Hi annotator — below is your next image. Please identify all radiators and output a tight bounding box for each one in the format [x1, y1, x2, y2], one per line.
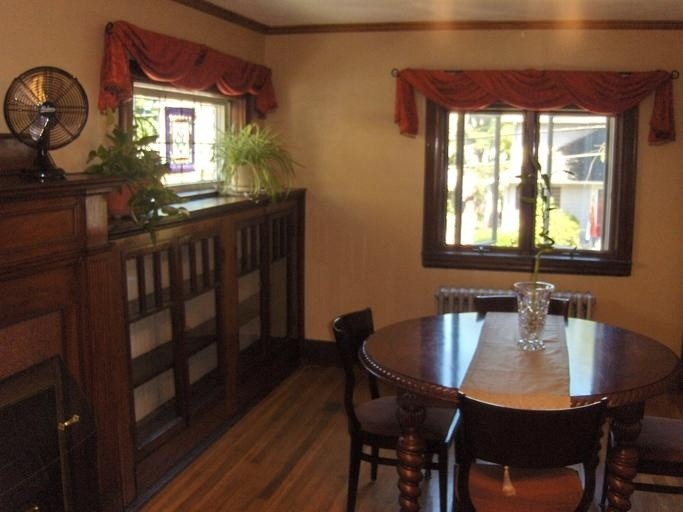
[433, 286, 598, 320]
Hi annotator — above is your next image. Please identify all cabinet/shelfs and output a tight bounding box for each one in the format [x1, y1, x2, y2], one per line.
[0, 174, 136, 510]
[122, 187, 309, 502]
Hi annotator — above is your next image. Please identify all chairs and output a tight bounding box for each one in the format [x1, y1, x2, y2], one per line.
[474, 294, 568, 316]
[454, 388, 607, 511]
[599, 413, 682, 512]
[332, 307, 464, 512]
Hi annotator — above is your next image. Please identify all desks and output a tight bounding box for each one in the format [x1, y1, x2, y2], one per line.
[358, 310, 683, 512]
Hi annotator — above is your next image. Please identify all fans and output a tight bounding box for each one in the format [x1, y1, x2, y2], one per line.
[3, 65, 89, 183]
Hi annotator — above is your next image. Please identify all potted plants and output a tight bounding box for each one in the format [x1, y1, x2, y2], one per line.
[514, 120, 562, 350]
[82, 101, 187, 246]
[208, 120, 307, 206]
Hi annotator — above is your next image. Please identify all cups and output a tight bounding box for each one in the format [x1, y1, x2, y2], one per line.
[513, 281, 555, 352]
